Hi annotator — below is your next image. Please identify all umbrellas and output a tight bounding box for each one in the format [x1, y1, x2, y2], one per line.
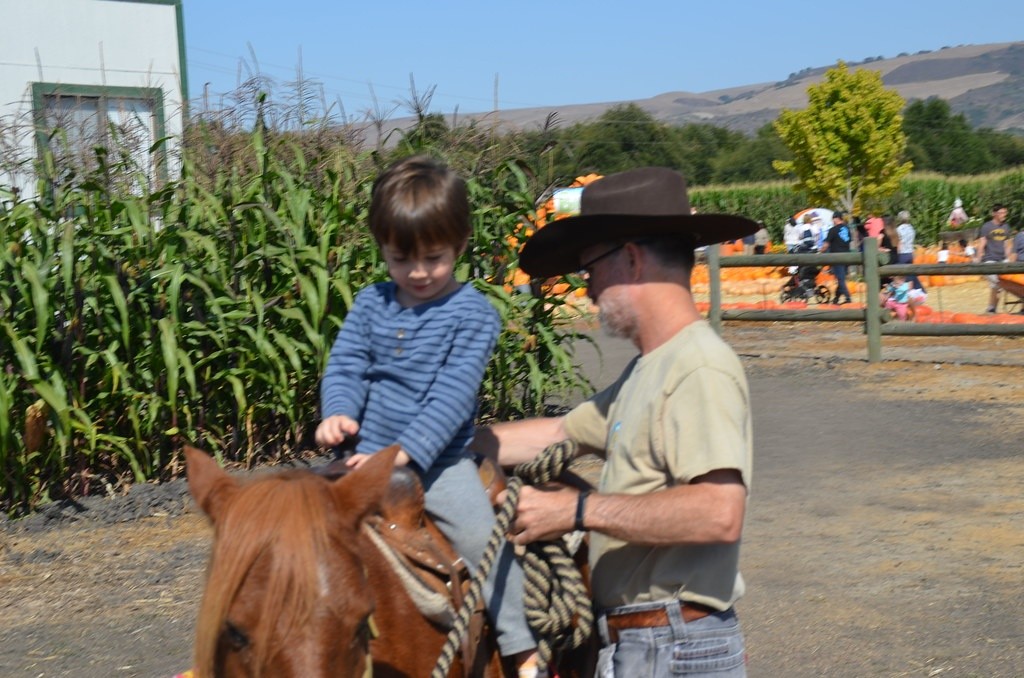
[786, 207, 836, 228]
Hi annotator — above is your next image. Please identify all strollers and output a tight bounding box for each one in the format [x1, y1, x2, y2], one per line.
[780, 240, 832, 304]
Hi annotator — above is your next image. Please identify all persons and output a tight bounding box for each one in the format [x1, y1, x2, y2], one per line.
[313, 153, 554, 678]
[755, 199, 1024, 322]
[470, 166, 764, 678]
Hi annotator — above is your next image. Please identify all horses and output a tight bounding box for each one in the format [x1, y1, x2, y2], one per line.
[183, 444, 599, 678]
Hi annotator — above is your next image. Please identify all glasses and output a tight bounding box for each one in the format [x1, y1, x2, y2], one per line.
[563, 248, 621, 286]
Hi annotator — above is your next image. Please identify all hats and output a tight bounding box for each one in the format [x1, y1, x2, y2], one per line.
[516, 166, 755, 279]
[833, 211, 843, 219]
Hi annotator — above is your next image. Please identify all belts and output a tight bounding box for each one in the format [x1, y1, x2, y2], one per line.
[606, 601, 716, 644]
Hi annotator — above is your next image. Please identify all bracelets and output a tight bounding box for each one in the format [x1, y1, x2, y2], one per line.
[576, 490, 592, 531]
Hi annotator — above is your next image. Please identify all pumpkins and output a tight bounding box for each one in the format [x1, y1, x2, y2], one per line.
[691, 242, 1024, 323]
[481, 174, 603, 295]
